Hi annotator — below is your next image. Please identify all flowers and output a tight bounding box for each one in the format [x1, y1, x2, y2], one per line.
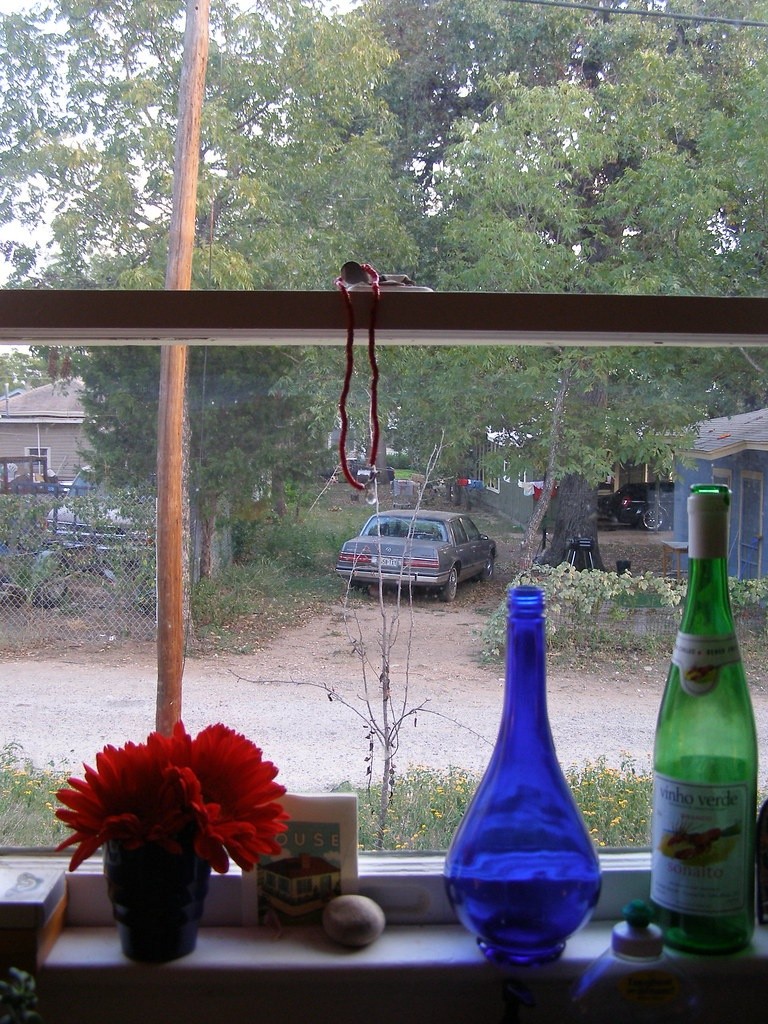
[55, 720, 291, 875]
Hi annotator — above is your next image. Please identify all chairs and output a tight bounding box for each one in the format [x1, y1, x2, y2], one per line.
[391, 480, 415, 510]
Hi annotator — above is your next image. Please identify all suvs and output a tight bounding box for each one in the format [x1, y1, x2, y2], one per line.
[608, 481, 674, 532]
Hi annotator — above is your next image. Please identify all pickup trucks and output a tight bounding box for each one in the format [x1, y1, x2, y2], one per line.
[319, 457, 395, 482]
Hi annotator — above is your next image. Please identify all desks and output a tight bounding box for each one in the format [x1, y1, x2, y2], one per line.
[661, 541, 688, 579]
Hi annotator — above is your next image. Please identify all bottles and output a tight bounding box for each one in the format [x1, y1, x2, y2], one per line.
[647, 484, 760, 956]
[442, 587, 603, 965]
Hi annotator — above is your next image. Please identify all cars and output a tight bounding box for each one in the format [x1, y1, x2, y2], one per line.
[335, 509, 497, 602]
[43, 463, 159, 568]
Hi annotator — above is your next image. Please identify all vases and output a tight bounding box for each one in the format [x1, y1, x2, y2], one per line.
[103, 841, 210, 965]
